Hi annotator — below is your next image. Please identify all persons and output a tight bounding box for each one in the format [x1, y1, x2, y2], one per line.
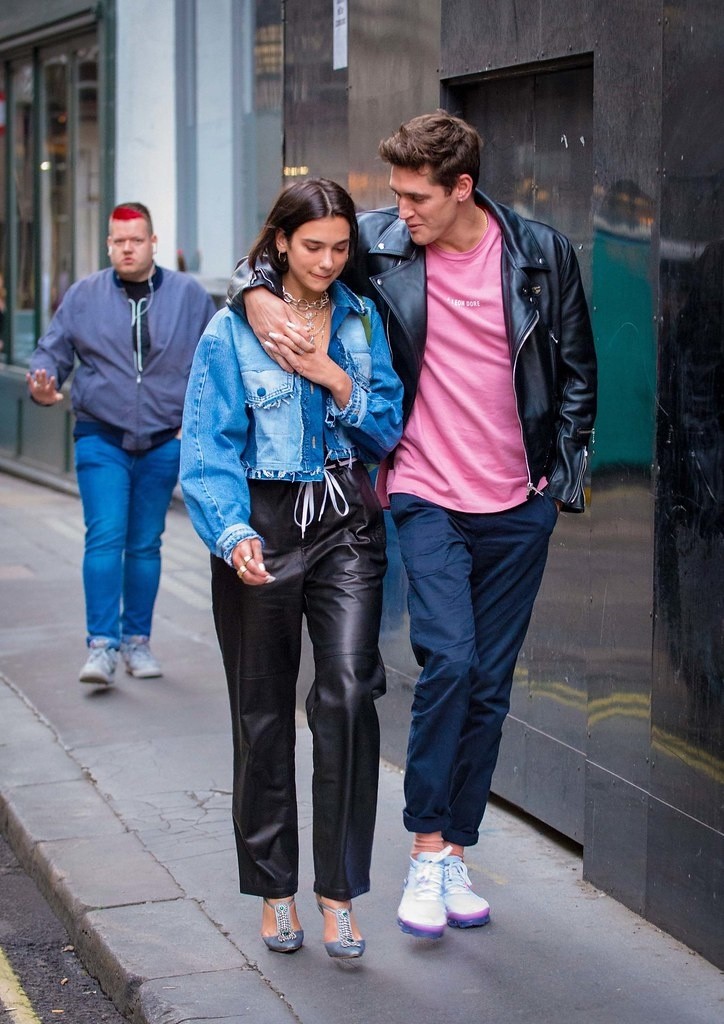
[177, 177, 403, 958]
[26, 203, 217, 683]
[225, 113, 598, 938]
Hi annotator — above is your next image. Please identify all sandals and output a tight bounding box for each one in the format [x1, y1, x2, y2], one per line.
[261, 894, 304, 953]
[314, 893, 366, 960]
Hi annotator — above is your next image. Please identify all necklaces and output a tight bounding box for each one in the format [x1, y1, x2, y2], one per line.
[283, 283, 329, 348]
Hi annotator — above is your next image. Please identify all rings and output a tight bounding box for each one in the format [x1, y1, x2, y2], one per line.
[244, 555, 251, 565]
[240, 566, 247, 573]
[237, 571, 243, 577]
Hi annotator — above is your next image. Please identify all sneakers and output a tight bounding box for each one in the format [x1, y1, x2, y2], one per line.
[396, 854, 448, 940]
[443, 854, 492, 928]
[118, 634, 165, 679]
[78, 642, 119, 685]
